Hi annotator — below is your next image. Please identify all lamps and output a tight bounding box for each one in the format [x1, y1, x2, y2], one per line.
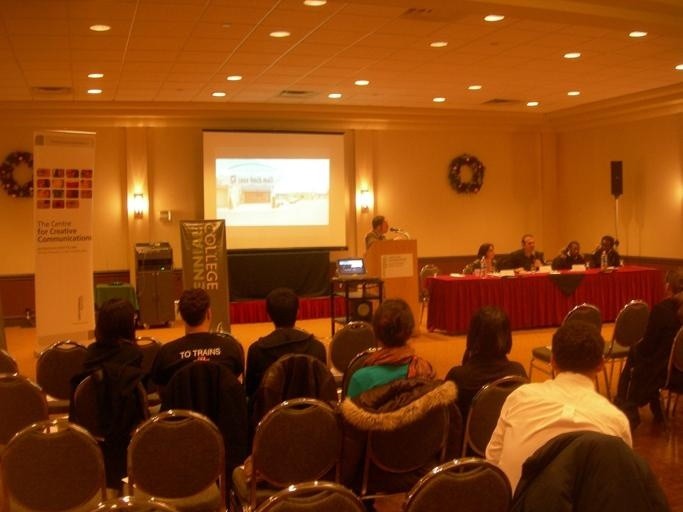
[352, 79, 370, 86]
[429, 40, 448, 48]
[565, 90, 581, 97]
[211, 90, 227, 97]
[327, 93, 343, 100]
[482, 14, 505, 23]
[86, 88, 103, 95]
[304, 0, 328, 7]
[225, 74, 243, 82]
[269, 31, 291, 38]
[672, 63, 683, 72]
[88, 22, 112, 33]
[561, 51, 582, 60]
[526, 100, 540, 108]
[87, 72, 104, 79]
[628, 30, 647, 39]
[467, 84, 481, 91]
[132, 193, 144, 220]
[359, 189, 370, 214]
[432, 96, 446, 104]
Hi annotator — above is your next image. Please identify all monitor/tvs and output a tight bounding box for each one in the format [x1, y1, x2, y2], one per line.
[338, 259, 366, 274]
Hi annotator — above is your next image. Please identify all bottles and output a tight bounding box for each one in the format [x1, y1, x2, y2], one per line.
[480, 256, 486, 277]
[601, 251, 607, 269]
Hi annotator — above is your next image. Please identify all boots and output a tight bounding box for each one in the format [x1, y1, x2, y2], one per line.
[651, 393, 667, 421]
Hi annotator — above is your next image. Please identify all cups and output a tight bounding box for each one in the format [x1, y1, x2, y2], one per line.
[531, 267, 536, 275]
[472, 269, 481, 278]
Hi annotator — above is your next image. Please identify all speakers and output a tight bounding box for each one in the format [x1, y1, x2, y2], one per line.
[610, 160, 624, 196]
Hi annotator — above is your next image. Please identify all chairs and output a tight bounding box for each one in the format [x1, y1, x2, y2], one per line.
[329, 321, 376, 384]
[360, 403, 450, 496]
[459, 374, 532, 474]
[602, 299, 649, 391]
[657, 325, 683, 418]
[254, 481, 366, 512]
[0, 373, 48, 445]
[136, 336, 161, 373]
[72, 370, 150, 442]
[160, 359, 246, 450]
[0, 420, 107, 512]
[127, 409, 226, 512]
[255, 354, 338, 416]
[229, 397, 341, 512]
[528, 304, 610, 401]
[402, 457, 512, 512]
[341, 347, 383, 399]
[35, 339, 88, 400]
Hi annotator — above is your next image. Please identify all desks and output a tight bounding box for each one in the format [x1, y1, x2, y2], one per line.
[424, 263, 665, 334]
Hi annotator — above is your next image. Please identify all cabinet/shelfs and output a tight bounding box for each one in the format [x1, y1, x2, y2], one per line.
[365, 237, 421, 330]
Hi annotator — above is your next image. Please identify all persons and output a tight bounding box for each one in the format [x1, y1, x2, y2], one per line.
[347, 299, 437, 399]
[552, 241, 585, 270]
[486, 321, 633, 496]
[613, 268, 683, 427]
[510, 234, 546, 271]
[365, 215, 389, 250]
[593, 236, 620, 267]
[83, 298, 159, 391]
[243, 287, 328, 398]
[444, 305, 528, 414]
[469, 242, 500, 274]
[151, 288, 245, 402]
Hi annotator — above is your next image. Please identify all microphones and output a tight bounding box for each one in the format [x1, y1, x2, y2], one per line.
[390, 227, 402, 231]
